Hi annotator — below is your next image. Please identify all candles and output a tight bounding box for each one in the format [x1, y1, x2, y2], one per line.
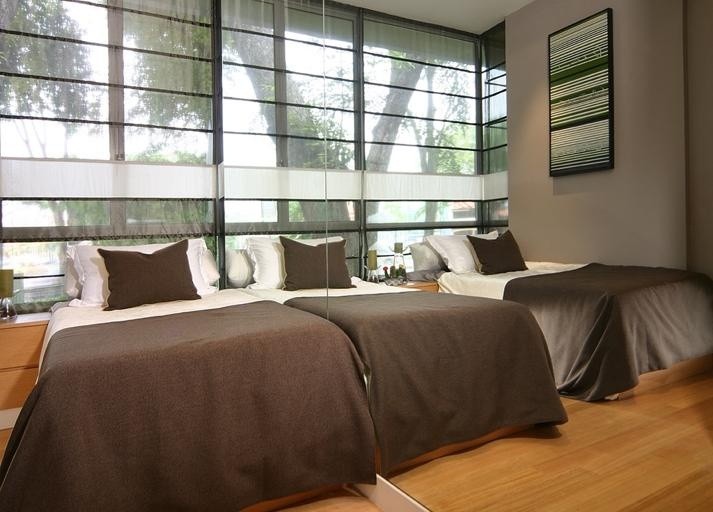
[0, 270, 13, 299]
[367, 250, 377, 270]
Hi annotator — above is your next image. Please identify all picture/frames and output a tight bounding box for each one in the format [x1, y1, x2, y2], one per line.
[548, 7, 614, 177]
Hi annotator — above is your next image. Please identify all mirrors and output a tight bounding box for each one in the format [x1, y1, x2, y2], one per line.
[211, 1, 713, 512]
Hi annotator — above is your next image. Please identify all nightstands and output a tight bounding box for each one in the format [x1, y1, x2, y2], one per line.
[0, 313, 51, 462]
[390, 279, 440, 293]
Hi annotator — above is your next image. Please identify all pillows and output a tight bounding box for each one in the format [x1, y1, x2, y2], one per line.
[226, 235, 356, 291]
[412, 229, 527, 275]
[61, 239, 222, 313]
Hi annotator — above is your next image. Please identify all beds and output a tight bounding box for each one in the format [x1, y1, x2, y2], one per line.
[442, 260, 713, 399]
[0, 292, 379, 512]
[228, 280, 568, 478]
